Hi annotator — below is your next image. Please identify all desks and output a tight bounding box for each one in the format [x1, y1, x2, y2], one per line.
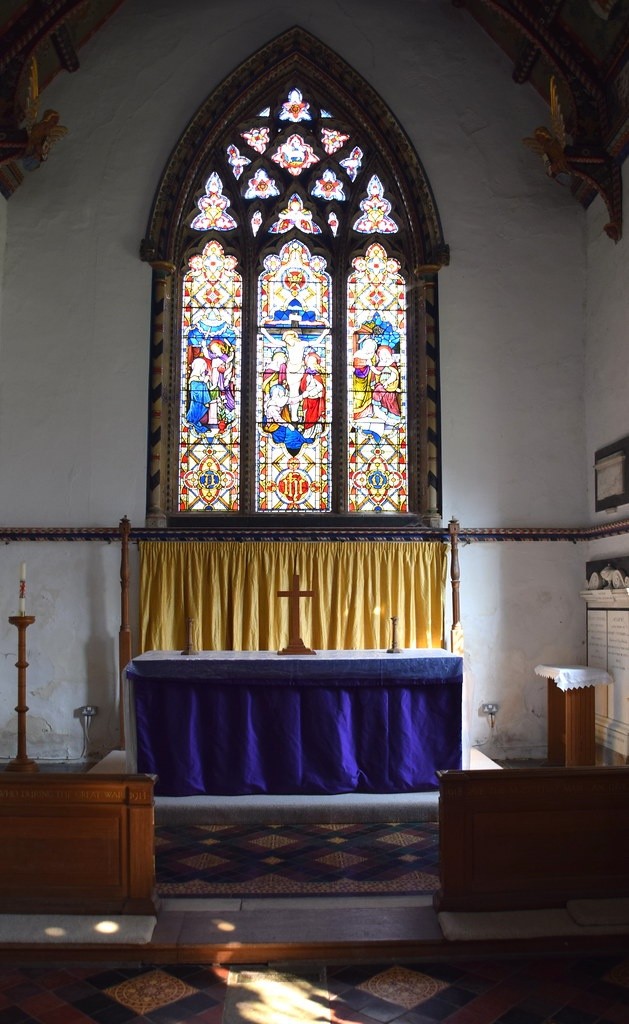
[122, 648, 464, 798]
[534, 665, 616, 768]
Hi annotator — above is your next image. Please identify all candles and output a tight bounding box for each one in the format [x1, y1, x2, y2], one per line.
[20, 561, 26, 612]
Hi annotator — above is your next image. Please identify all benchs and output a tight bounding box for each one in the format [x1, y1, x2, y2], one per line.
[0, 771, 160, 943]
[434, 769, 629, 940]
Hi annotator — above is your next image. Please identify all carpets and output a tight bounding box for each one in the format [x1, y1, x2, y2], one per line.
[154, 823, 442, 897]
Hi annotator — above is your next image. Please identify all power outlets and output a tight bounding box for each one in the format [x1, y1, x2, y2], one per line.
[482, 704, 499, 713]
[83, 705, 97, 715]
